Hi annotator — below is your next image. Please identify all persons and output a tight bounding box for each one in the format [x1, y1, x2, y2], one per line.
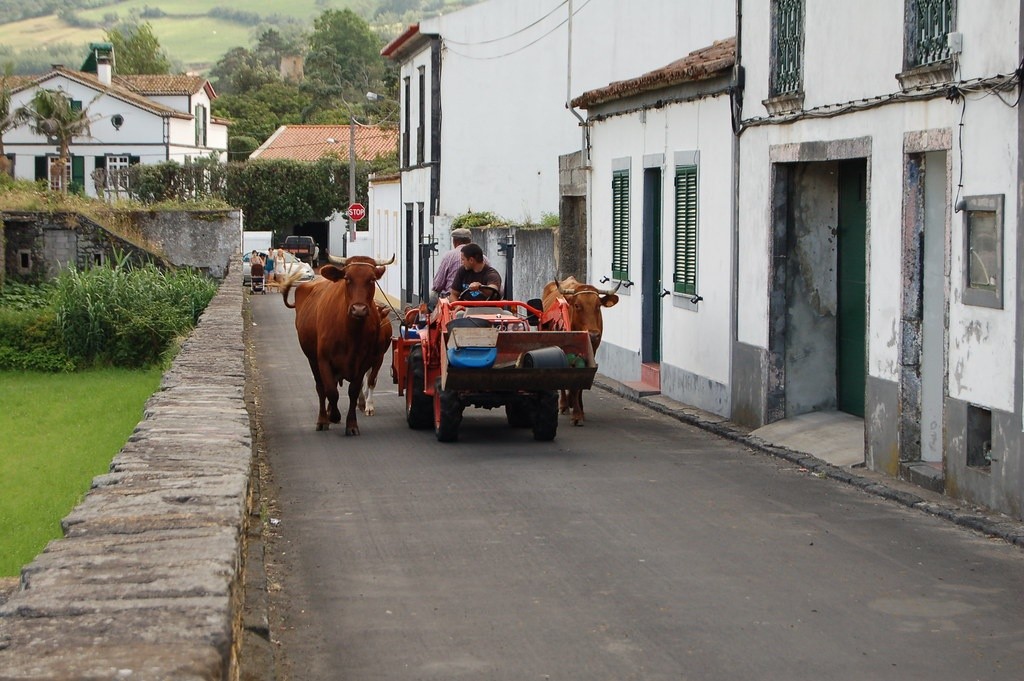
[264, 248, 276, 293]
[250, 250, 264, 294]
[275, 249, 286, 293]
[312, 243, 319, 269]
[427, 228, 491, 315]
[449, 244, 501, 319]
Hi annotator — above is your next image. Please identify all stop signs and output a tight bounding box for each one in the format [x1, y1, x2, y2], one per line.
[348, 202, 366, 222]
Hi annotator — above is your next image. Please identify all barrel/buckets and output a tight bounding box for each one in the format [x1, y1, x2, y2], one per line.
[445, 346, 499, 368]
[523, 346, 569, 369]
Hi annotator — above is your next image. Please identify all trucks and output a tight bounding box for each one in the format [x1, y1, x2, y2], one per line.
[280, 235, 320, 268]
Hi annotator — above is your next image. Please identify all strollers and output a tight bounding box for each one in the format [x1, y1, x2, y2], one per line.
[249, 263, 267, 295]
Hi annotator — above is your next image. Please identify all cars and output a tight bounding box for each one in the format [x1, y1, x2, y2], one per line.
[242, 249, 315, 286]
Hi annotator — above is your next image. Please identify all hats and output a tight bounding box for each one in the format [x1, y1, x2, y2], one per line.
[451, 228, 471, 238]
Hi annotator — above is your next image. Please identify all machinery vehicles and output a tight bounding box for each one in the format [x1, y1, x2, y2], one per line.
[389, 235, 599, 443]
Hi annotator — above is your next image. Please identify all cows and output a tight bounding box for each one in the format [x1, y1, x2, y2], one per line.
[266, 253, 398, 437]
[541, 275, 622, 427]
